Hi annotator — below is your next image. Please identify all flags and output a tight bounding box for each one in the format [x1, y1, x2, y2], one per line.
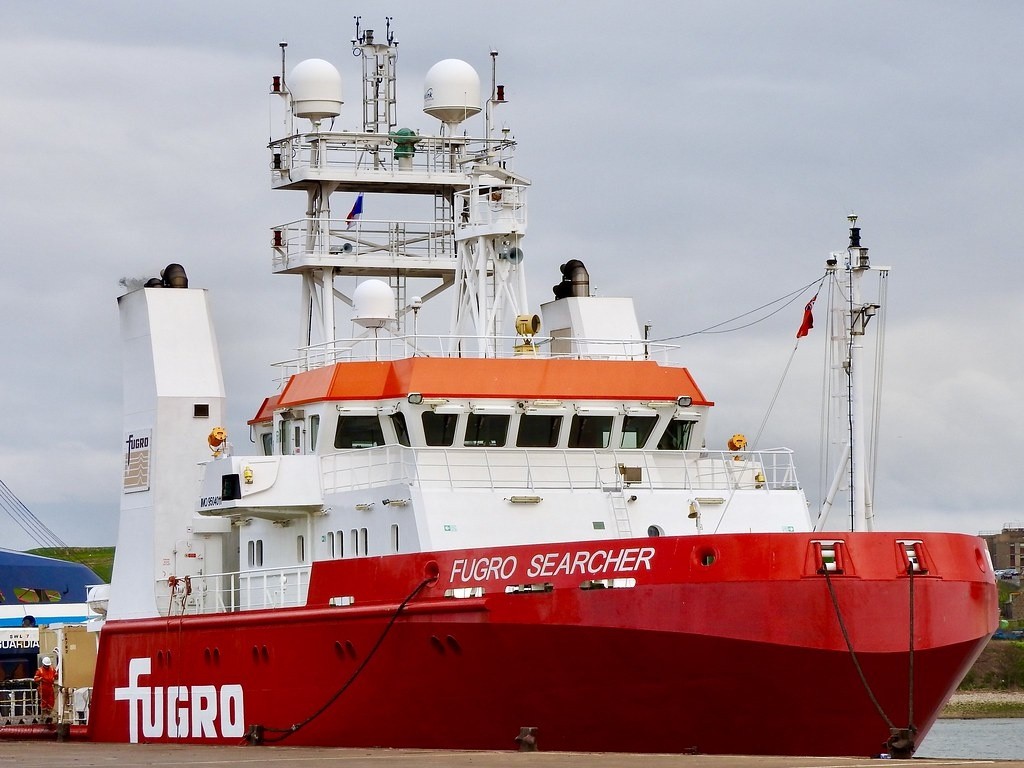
[345, 192, 363, 230]
[796, 292, 819, 338]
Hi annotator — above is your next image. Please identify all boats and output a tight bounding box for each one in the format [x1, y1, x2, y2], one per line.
[0, 11, 1001, 755]
[0, 480, 108, 688]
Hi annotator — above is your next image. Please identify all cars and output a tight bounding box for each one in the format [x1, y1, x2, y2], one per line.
[994, 568, 1020, 580]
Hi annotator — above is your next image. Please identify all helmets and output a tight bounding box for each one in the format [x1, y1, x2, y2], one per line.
[42, 657, 52, 666]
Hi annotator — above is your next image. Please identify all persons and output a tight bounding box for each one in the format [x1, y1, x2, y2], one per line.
[34, 657, 58, 723]
